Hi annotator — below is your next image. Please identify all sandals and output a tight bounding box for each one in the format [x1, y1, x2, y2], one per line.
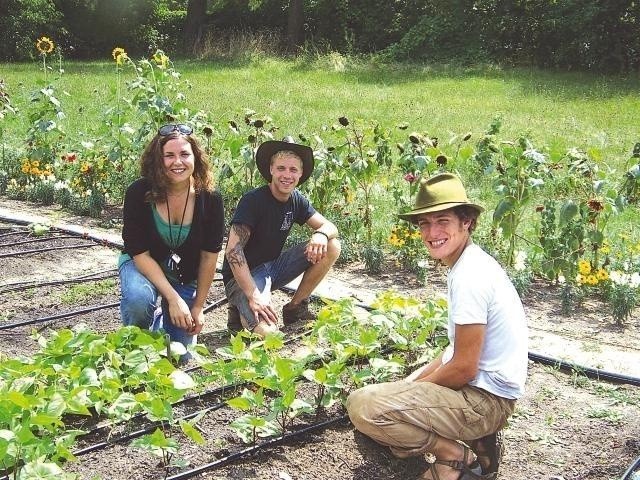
[472, 427, 505, 478]
[416, 444, 482, 480]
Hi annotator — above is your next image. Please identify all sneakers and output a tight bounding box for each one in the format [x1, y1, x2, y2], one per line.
[281, 300, 319, 326]
[226, 304, 243, 333]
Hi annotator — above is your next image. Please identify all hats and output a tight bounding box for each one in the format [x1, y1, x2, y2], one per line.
[255, 139, 315, 189]
[395, 171, 487, 222]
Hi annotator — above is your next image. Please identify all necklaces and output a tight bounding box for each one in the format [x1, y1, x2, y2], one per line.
[163, 183, 192, 273]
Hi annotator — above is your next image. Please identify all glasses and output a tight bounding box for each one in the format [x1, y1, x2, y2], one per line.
[157, 123, 193, 136]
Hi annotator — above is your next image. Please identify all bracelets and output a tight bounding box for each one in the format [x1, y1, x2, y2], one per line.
[310, 231, 330, 240]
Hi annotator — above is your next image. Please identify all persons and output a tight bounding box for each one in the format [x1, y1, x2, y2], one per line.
[339, 171, 526, 480]
[116, 123, 227, 356]
[218, 132, 343, 341]
